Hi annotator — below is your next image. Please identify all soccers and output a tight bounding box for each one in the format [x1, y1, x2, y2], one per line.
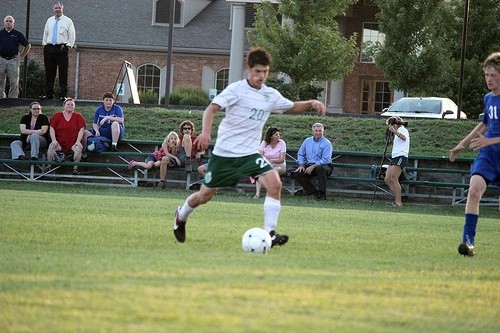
[242, 227, 272, 254]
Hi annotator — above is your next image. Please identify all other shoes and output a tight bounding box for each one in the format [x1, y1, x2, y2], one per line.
[459, 243, 475, 256]
[45, 95, 53, 99]
[60, 96, 66, 100]
[111, 145, 118, 151]
[39, 167, 51, 173]
[317, 195, 326, 200]
[315, 192, 321, 198]
[73, 169, 80, 174]
[185, 162, 192, 173]
[81, 157, 87, 161]
[158, 182, 165, 189]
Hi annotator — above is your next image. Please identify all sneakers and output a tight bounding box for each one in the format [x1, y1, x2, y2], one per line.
[173, 206, 186, 242]
[270, 230, 288, 248]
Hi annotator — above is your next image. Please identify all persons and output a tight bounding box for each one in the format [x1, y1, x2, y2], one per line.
[292, 123, 335, 200]
[39, 2, 76, 100]
[253, 128, 287, 199]
[128, 120, 208, 189]
[449, 53, 500, 255]
[10, 102, 51, 161]
[83, 92, 126, 159]
[45, 97, 86, 175]
[173, 48, 326, 248]
[384, 116, 409, 208]
[0, 16, 31, 98]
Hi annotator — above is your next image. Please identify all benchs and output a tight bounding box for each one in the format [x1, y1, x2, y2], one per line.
[0, 132, 500, 208]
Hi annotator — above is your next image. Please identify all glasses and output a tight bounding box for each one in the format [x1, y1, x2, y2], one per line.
[32, 108, 40, 110]
[183, 128, 192, 130]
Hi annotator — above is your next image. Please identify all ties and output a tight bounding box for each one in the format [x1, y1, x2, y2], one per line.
[52, 19, 60, 45]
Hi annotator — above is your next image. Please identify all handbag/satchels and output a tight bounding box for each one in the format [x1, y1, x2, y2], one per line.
[85, 136, 111, 153]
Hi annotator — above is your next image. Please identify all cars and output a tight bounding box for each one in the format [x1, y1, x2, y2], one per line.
[380, 96, 468, 121]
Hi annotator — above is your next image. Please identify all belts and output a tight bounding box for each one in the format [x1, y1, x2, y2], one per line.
[2, 56, 15, 60]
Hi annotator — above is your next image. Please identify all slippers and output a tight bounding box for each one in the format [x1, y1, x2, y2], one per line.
[387, 202, 402, 207]
[128, 160, 134, 169]
